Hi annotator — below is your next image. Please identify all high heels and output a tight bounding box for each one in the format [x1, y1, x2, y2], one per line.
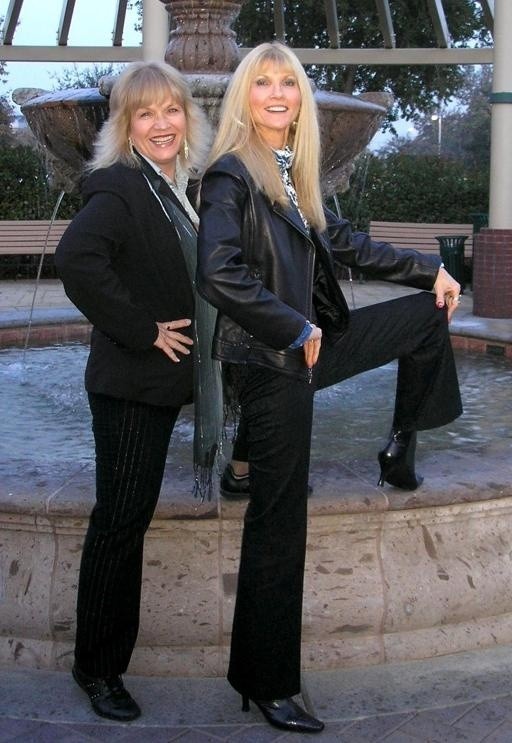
[374, 428, 425, 490]
[240, 688, 326, 733]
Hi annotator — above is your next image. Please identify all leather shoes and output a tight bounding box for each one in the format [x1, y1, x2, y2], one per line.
[71, 665, 141, 720]
[220, 465, 313, 500]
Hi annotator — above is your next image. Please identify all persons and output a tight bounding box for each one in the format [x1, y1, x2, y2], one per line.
[195, 40, 463, 735]
[54, 59, 221, 721]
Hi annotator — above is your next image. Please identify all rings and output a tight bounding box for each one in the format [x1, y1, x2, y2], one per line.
[450, 294, 462, 305]
[165, 322, 170, 330]
[310, 338, 315, 342]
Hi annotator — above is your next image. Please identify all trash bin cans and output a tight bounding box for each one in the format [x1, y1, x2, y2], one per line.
[435, 235, 469, 293]
